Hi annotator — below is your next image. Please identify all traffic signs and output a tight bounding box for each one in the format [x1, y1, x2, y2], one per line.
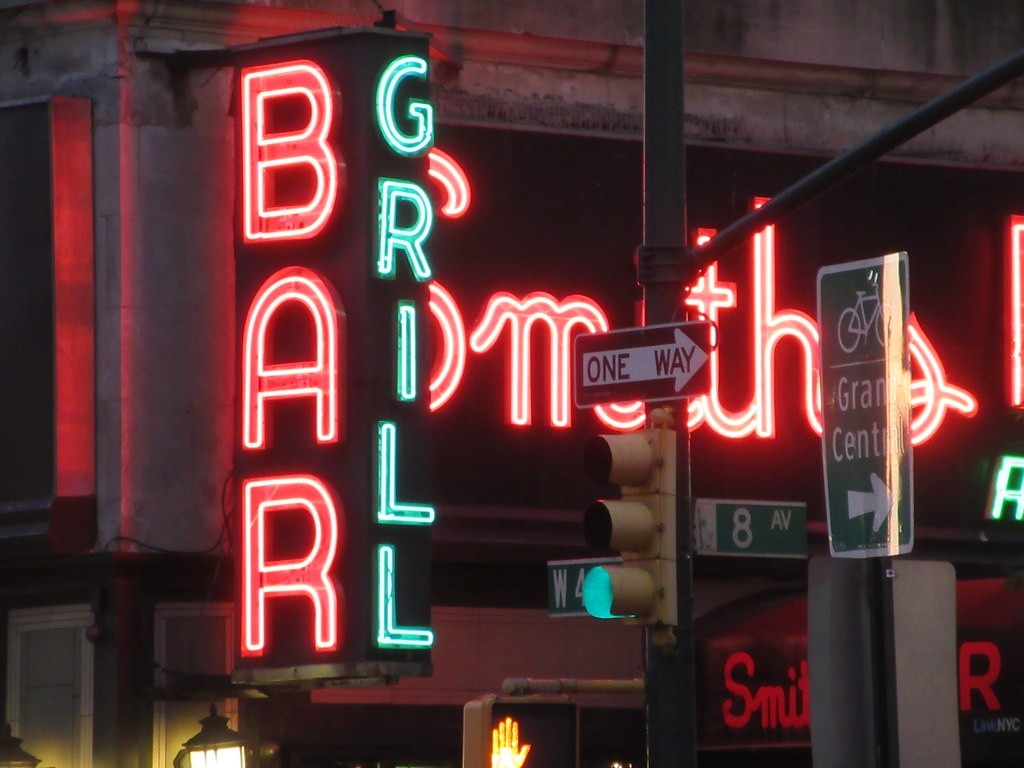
[817, 251, 910, 559]
[573, 320, 713, 408]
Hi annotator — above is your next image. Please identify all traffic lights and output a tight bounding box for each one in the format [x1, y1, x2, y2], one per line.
[580, 424, 688, 631]
[462, 695, 582, 768]
